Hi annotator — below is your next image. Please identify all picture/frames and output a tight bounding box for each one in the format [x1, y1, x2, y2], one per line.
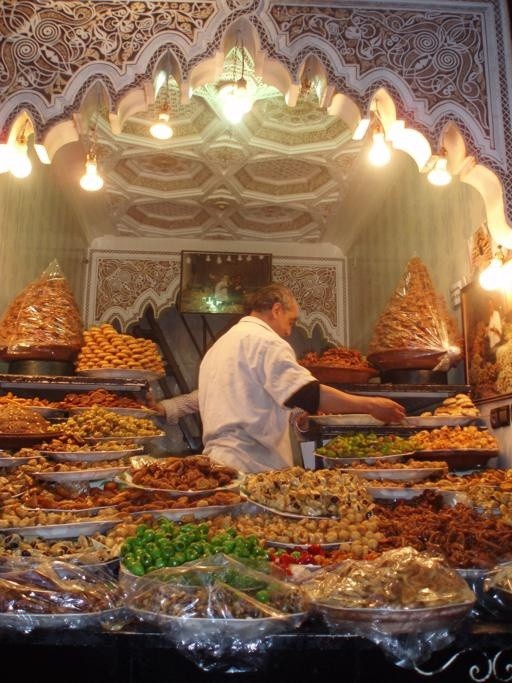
[180, 250, 272, 316]
[458, 269, 511, 405]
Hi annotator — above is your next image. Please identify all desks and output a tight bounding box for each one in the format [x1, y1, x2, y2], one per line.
[0, 622, 512, 681]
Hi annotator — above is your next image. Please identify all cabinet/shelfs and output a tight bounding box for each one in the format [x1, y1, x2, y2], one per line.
[0, 374, 156, 467]
[314, 383, 473, 474]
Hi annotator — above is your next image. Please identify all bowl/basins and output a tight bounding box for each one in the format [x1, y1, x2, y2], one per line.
[1, 343, 79, 362]
[456, 567, 489, 581]
[303, 364, 375, 386]
[128, 602, 307, 635]
[471, 567, 511, 623]
[437, 489, 474, 511]
[366, 349, 445, 370]
[291, 563, 322, 580]
[314, 599, 478, 636]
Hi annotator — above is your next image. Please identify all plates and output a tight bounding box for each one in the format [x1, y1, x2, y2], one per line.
[5, 370, 500, 632]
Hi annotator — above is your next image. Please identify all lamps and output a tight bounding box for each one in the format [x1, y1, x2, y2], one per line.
[480, 244, 505, 267]
[428, 121, 451, 186]
[149, 103, 173, 140]
[215, 78, 241, 126]
[234, 49, 254, 113]
[368, 132, 390, 166]
[8, 119, 33, 179]
[80, 152, 103, 192]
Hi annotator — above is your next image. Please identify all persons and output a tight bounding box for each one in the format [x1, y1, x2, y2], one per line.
[215, 273, 232, 296]
[197, 284, 406, 473]
[209, 272, 222, 290]
[153, 387, 325, 468]
[229, 274, 247, 296]
